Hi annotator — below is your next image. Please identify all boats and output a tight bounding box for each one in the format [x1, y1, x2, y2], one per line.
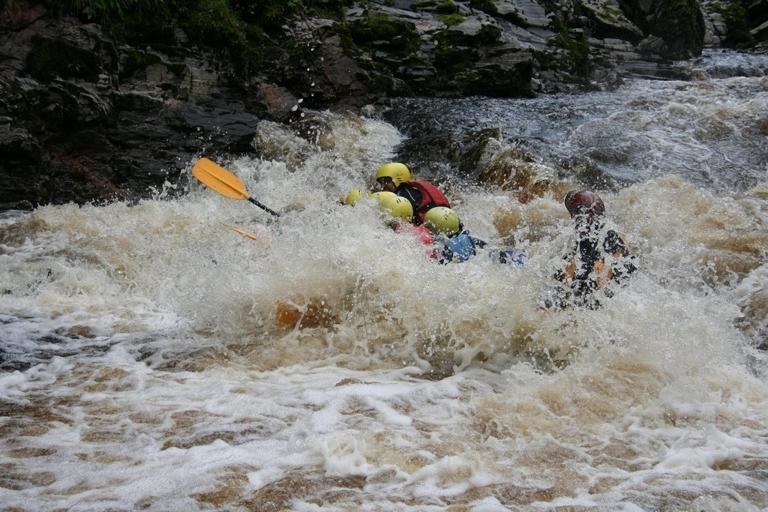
[272, 255, 611, 328]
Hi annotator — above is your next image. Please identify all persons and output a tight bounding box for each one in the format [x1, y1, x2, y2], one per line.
[344, 162, 528, 274]
[539, 187, 640, 319]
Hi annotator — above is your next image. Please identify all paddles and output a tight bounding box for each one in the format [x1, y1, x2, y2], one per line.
[222, 221, 258, 242]
[191, 157, 279, 216]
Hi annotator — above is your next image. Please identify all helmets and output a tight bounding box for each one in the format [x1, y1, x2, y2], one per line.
[375, 162, 413, 189]
[423, 206, 460, 237]
[344, 189, 414, 227]
[565, 189, 605, 225]
